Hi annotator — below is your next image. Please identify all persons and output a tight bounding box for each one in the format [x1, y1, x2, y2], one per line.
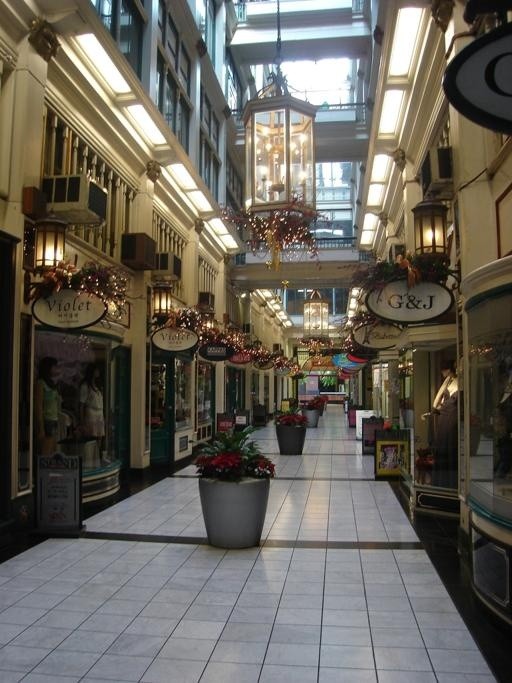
[34, 357, 108, 466]
[151, 369, 164, 418]
[482, 360, 512, 478]
[421, 360, 461, 479]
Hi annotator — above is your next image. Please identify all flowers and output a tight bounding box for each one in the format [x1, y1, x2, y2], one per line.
[275, 410, 308, 426]
[299, 394, 327, 410]
[192, 424, 273, 480]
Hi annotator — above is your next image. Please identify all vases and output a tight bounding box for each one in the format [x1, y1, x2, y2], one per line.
[302, 409, 318, 426]
[197, 474, 269, 550]
[275, 423, 305, 454]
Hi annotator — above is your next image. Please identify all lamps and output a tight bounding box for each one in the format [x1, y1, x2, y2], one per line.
[145, 279, 174, 334]
[406, 188, 461, 293]
[299, 283, 333, 365]
[22, 206, 67, 273]
[222, 0, 319, 268]
